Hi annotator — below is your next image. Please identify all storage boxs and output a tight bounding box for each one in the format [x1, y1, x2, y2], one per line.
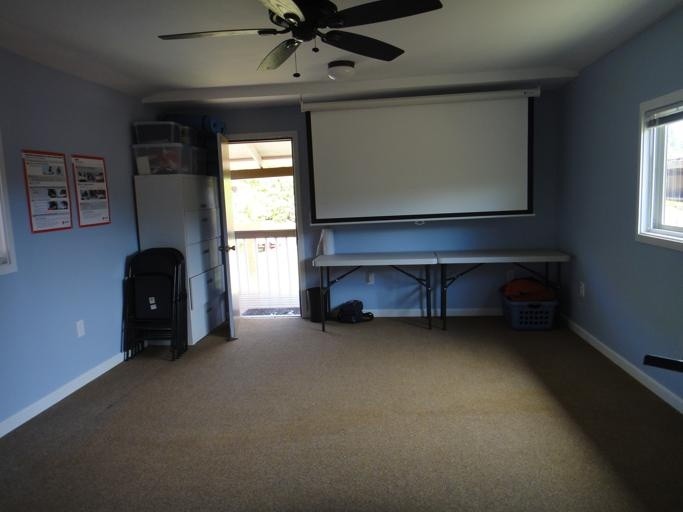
[130, 120, 199, 173]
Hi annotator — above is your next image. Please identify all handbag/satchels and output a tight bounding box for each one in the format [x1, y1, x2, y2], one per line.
[338, 297, 373, 324]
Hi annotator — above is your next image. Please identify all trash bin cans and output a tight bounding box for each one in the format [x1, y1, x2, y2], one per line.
[306, 287, 327, 322]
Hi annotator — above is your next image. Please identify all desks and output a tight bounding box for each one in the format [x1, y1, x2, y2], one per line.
[432, 251, 569, 331]
[310, 250, 438, 332]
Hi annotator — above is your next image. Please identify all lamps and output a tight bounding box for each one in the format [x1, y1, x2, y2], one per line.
[327, 61, 354, 80]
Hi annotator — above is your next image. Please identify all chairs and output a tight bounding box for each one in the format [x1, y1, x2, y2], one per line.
[123, 245, 188, 360]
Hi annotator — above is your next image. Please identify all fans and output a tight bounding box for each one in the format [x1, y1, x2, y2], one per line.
[152, 1, 441, 71]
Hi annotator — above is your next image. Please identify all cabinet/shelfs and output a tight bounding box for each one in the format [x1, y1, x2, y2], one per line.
[132, 173, 224, 345]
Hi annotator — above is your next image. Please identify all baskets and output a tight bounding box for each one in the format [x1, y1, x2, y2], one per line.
[499, 282, 556, 331]
